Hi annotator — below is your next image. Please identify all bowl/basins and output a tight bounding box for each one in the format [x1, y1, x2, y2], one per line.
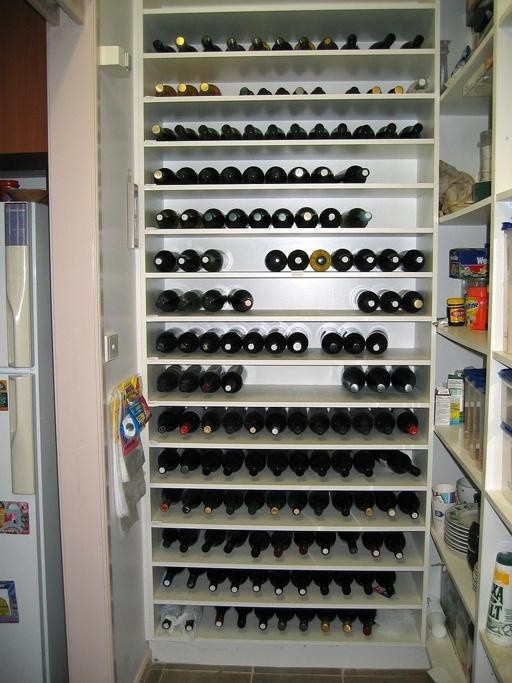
[456, 476, 477, 502]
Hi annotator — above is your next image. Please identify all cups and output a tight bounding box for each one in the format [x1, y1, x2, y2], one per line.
[432, 485, 456, 534]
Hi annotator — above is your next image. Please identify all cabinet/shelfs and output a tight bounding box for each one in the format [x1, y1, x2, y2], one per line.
[133, 1, 442, 670]
[428, 1, 512, 682]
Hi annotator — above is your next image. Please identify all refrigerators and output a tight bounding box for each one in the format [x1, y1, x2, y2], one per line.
[1, 200, 69, 682]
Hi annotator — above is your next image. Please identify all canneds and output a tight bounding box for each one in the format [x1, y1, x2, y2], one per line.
[447, 298, 466, 326]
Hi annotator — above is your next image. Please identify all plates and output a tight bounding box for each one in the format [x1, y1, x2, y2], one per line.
[444, 502, 478, 555]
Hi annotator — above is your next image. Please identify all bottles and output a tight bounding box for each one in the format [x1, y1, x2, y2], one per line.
[159, 605, 205, 632]
[478, 130, 491, 183]
[152, 122, 423, 141]
[156, 448, 423, 478]
[152, 164, 370, 185]
[156, 287, 253, 313]
[157, 488, 422, 520]
[346, 78, 426, 94]
[154, 81, 223, 96]
[153, 249, 230, 272]
[440, 40, 451, 94]
[340, 366, 416, 397]
[240, 86, 326, 95]
[153, 207, 373, 230]
[157, 407, 419, 436]
[465, 277, 488, 330]
[162, 565, 398, 596]
[150, 34, 426, 54]
[426, 595, 447, 638]
[264, 248, 425, 272]
[154, 363, 249, 393]
[355, 288, 425, 314]
[485, 550, 512, 647]
[161, 528, 407, 561]
[211, 606, 378, 637]
